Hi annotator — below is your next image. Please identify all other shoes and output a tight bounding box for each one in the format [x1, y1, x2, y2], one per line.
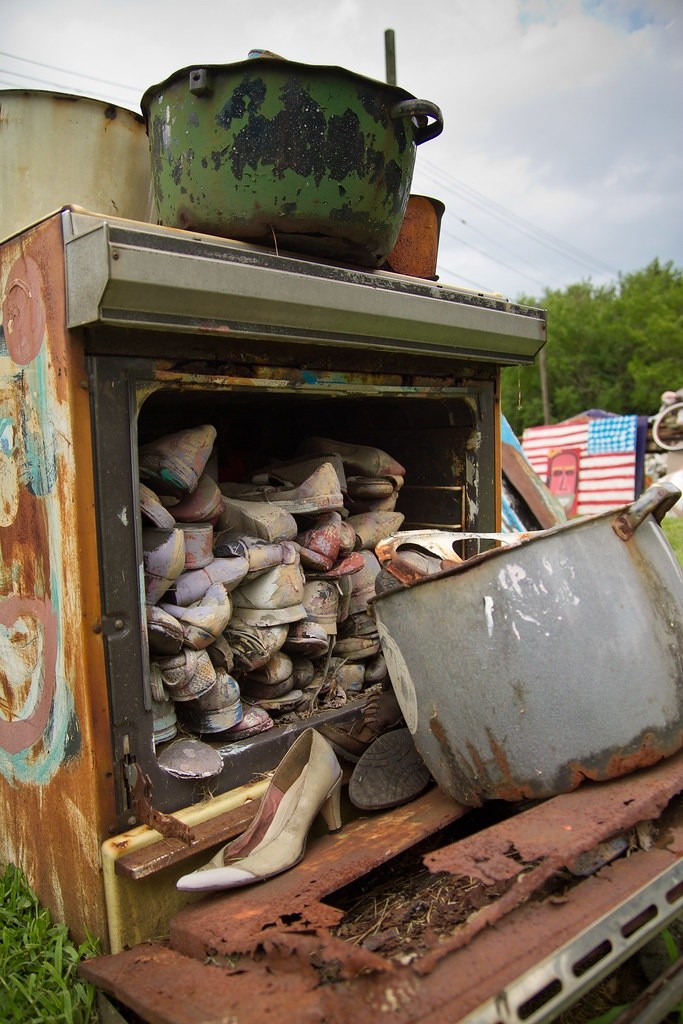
[134, 424, 446, 782]
[340, 732, 426, 811]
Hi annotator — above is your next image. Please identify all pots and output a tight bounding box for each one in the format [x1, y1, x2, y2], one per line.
[366, 480, 682, 808]
[139, 56, 443, 270]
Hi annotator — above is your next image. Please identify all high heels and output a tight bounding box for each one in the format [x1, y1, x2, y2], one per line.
[176, 724, 344, 894]
